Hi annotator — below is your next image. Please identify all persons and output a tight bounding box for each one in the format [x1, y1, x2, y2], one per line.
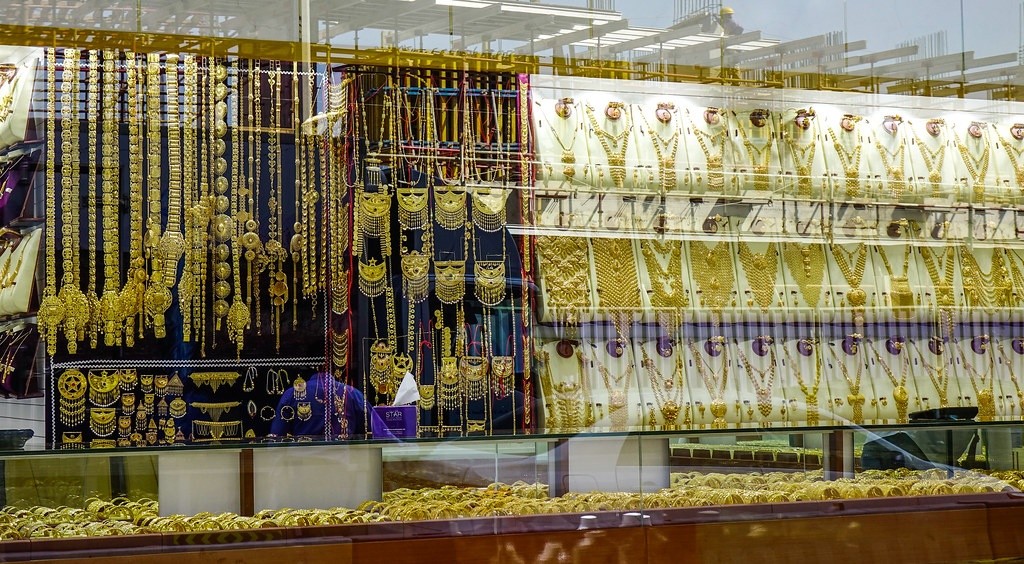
[270, 329, 395, 438]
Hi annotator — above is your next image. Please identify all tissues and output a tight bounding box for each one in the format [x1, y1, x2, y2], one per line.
[371, 371, 422, 441]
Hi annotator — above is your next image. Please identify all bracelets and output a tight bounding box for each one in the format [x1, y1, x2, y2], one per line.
[0, 466, 1024, 540]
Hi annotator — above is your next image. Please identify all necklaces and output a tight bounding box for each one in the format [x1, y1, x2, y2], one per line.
[37, 48, 1024, 430]
[0, 67, 32, 384]
